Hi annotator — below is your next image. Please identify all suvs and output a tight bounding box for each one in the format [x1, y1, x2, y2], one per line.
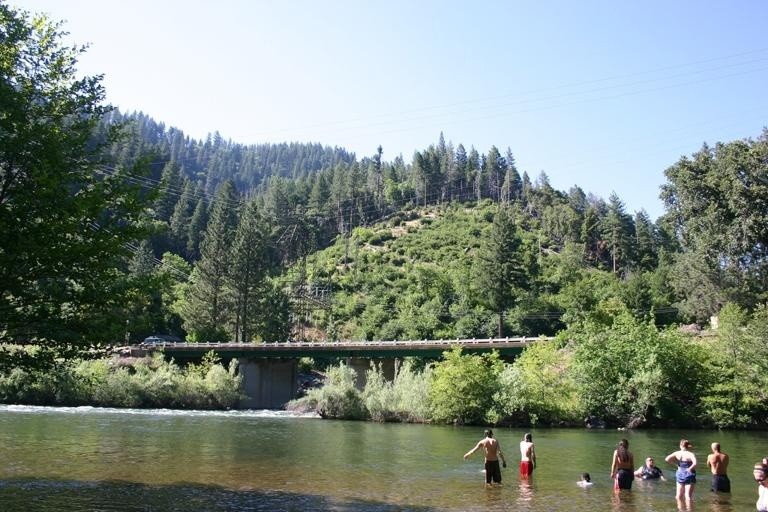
[143, 335, 172, 347]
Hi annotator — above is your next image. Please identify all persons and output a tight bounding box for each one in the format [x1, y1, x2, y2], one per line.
[706, 440, 733, 495]
[610, 437, 635, 490]
[664, 439, 697, 498]
[575, 471, 597, 488]
[633, 456, 667, 482]
[751, 462, 768, 512]
[462, 428, 506, 488]
[518, 431, 538, 485]
[761, 455, 768, 467]
[675, 498, 694, 511]
